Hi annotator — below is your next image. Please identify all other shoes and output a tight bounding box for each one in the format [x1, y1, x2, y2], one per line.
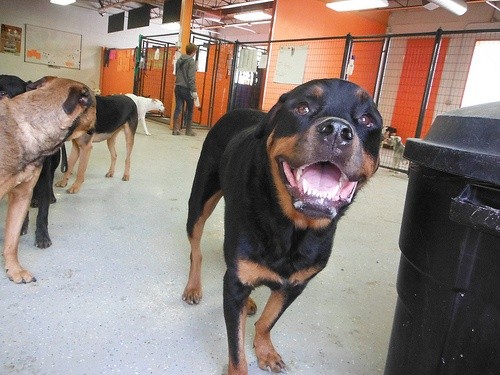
[185, 121, 197, 136]
[172, 128, 182, 135]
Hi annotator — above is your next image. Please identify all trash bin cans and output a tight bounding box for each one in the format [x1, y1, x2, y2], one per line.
[381, 101, 500, 375]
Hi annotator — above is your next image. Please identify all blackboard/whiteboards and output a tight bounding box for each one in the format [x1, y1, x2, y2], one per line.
[22, 24, 82, 72]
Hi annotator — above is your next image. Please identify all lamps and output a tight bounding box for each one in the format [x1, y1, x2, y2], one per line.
[233, 11, 272, 22]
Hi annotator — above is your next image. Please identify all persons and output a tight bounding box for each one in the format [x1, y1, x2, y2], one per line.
[172, 43, 198, 136]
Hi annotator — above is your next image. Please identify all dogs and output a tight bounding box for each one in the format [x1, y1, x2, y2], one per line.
[181, 78, 383, 375]
[122, 94, 166, 136]
[54, 94, 138, 194]
[0, 75, 68, 249]
[387, 136, 412, 176]
[0, 75, 96, 284]
